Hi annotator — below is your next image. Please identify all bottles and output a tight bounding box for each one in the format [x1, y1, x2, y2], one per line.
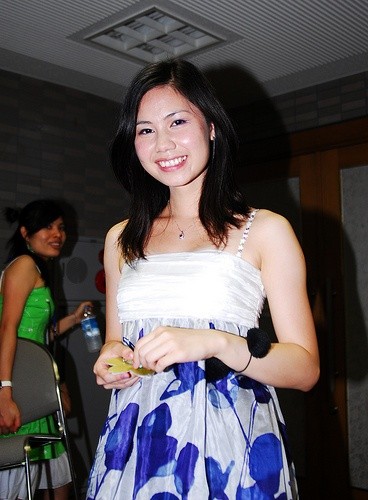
[79, 304, 103, 354]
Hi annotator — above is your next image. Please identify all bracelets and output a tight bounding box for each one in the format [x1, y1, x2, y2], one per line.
[0, 380, 13, 388]
[236, 327, 271, 374]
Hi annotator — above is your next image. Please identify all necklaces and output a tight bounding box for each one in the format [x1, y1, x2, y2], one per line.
[168, 200, 195, 237]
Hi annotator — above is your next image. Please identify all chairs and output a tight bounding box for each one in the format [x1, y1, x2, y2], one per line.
[0, 338, 80, 500]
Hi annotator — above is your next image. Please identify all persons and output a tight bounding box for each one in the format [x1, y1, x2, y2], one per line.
[0, 198, 95, 500]
[74, 57, 320, 500]
